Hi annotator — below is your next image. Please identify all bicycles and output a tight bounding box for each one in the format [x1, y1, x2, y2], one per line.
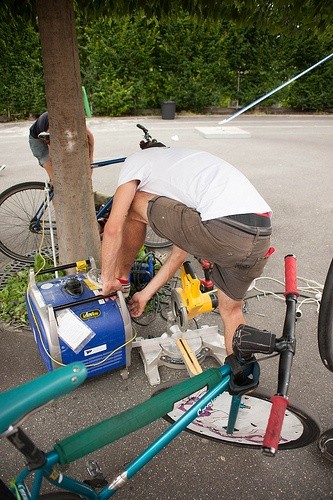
[0, 253, 321, 500]
[0, 123, 177, 266]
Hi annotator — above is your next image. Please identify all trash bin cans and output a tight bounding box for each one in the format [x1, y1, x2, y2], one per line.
[162, 101, 176, 119]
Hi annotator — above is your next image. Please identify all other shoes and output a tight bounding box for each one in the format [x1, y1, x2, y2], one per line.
[87, 268, 133, 299]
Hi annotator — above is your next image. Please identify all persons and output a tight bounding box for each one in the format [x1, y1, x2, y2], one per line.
[99, 139, 273, 392]
[28, 101, 96, 211]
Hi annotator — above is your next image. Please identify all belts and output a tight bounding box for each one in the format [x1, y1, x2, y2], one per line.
[229, 213, 272, 227]
